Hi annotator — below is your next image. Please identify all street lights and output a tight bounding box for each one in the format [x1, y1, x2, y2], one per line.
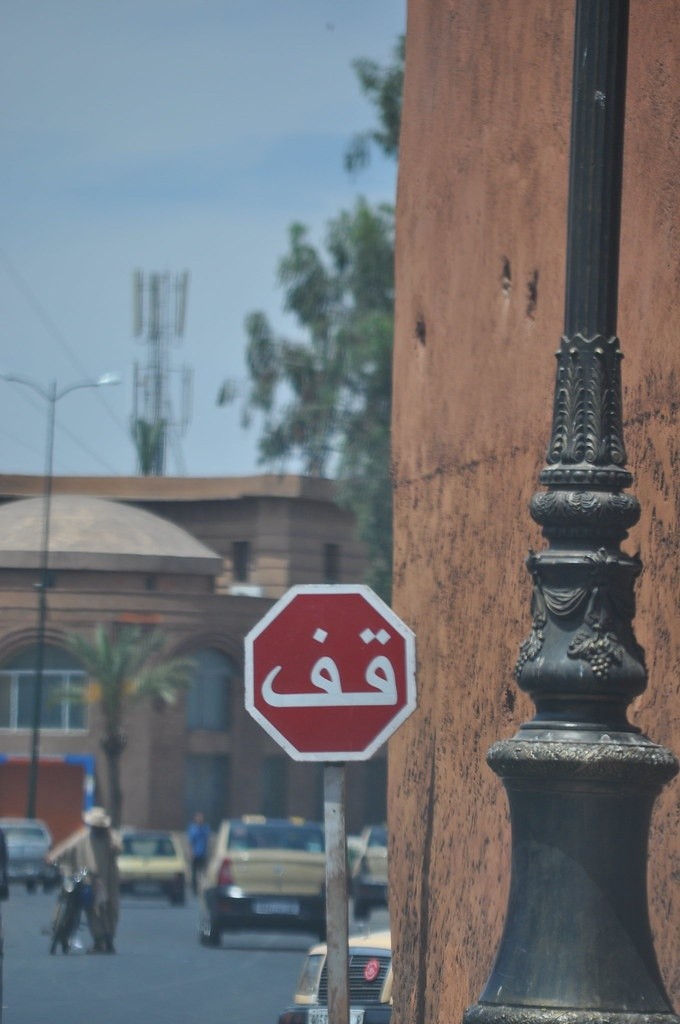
[0, 370, 126, 816]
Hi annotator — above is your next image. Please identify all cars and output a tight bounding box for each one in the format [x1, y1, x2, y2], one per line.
[117, 828, 191, 908]
[200, 814, 331, 945]
[285, 926, 396, 1023]
[353, 825, 399, 918]
[0, 822, 60, 901]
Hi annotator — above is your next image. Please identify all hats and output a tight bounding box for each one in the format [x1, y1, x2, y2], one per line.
[83, 807, 111, 828]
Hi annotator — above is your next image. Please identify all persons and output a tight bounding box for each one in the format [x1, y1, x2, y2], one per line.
[47, 806, 122, 956]
[188, 812, 211, 898]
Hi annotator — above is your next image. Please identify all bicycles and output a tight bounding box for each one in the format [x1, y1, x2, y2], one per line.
[46, 866, 91, 955]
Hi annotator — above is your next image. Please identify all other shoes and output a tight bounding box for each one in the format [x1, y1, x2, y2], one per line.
[94, 935, 115, 955]
